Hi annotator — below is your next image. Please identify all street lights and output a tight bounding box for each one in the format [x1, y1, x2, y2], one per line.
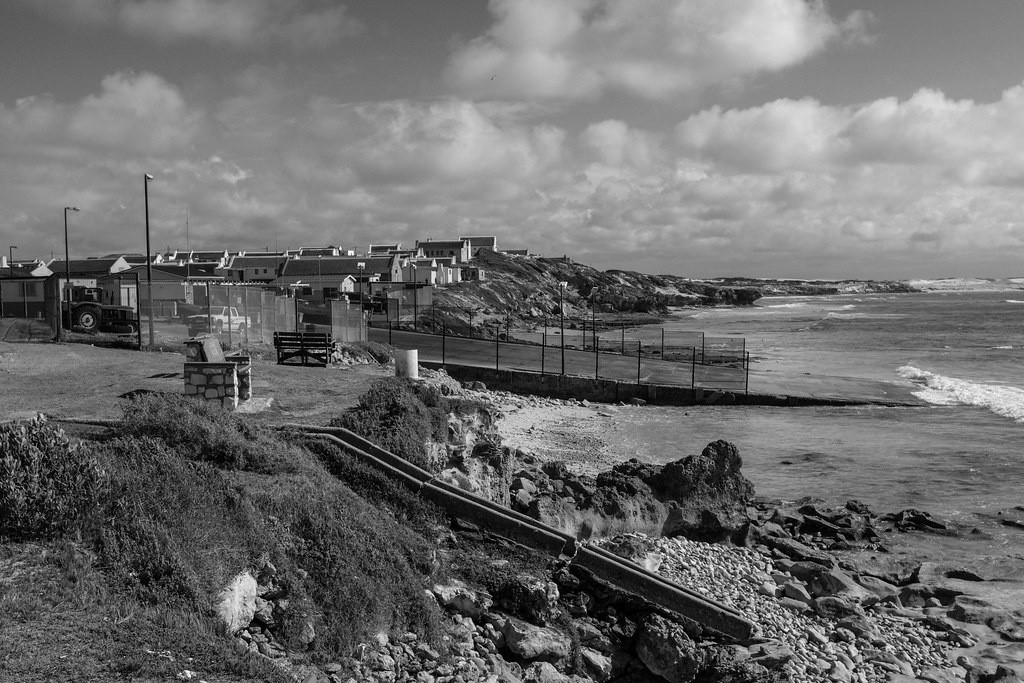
[357, 262, 366, 341]
[591, 286, 599, 352]
[65, 207, 81, 330]
[410, 263, 418, 330]
[144, 173, 155, 344]
[9, 246, 18, 277]
[558, 281, 568, 374]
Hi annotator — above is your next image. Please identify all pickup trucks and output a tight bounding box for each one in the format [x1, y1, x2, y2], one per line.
[186, 306, 251, 338]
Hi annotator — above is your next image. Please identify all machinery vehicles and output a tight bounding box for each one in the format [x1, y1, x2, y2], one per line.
[65, 287, 137, 334]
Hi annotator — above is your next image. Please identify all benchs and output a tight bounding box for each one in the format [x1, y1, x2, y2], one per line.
[183, 338, 252, 408]
[274, 331, 337, 368]
